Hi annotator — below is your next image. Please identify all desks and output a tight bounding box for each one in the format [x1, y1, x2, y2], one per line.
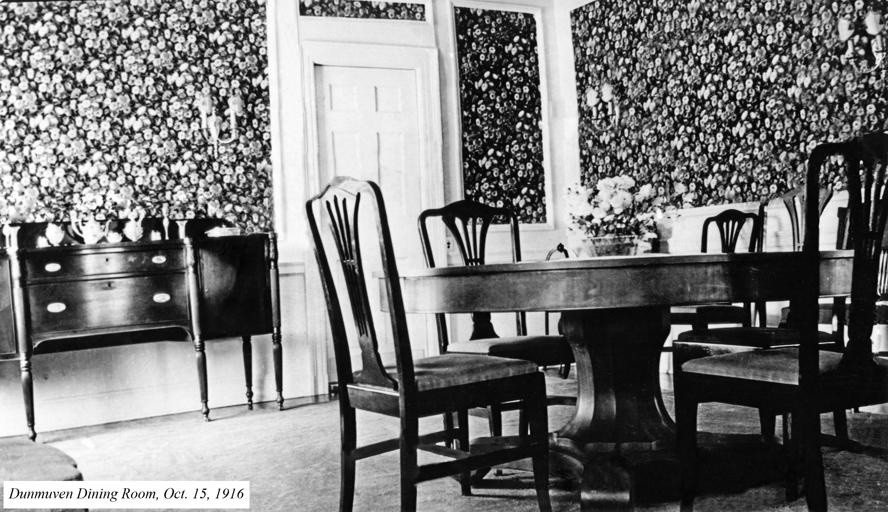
[0, 219, 283, 444]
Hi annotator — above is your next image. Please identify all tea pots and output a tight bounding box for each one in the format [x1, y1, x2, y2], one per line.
[71, 213, 113, 245]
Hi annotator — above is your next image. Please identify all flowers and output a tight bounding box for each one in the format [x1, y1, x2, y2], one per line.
[564, 176, 665, 239]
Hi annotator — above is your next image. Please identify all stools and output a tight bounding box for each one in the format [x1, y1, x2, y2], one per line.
[0, 442, 84, 511]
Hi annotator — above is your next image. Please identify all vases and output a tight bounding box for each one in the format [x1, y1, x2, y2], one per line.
[587, 234, 638, 257]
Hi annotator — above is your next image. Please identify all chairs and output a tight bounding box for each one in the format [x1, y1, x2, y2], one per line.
[659, 208, 759, 385]
[777, 198, 888, 338]
[305, 175, 554, 510]
[777, 183, 837, 250]
[417, 199, 574, 482]
[679, 129, 888, 512]
[672, 183, 849, 455]
